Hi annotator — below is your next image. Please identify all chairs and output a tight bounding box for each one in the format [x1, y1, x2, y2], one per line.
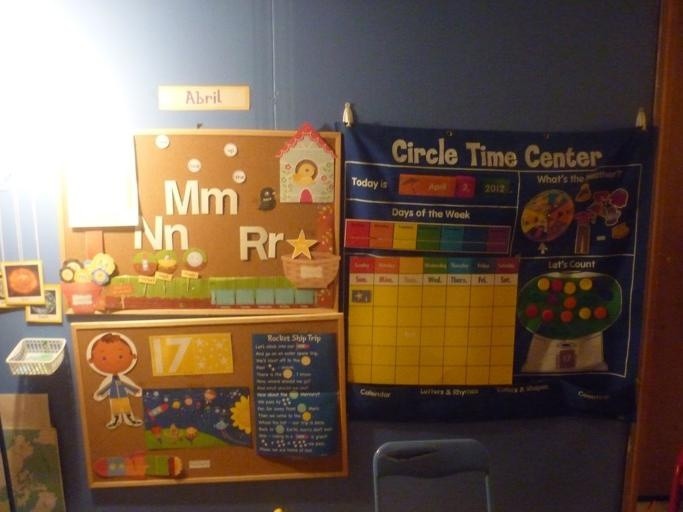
[372, 439, 493, 512]
